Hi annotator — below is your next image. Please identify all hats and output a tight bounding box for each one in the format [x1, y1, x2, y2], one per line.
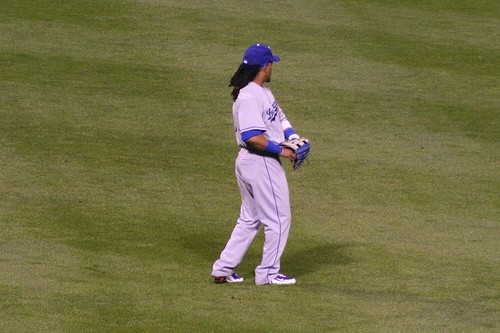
[242, 44, 280, 65]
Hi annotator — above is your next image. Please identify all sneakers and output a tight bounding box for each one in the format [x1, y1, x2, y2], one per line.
[212, 273, 243, 283]
[267, 275, 296, 286]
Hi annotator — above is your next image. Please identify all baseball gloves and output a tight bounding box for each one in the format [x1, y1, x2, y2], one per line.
[277, 137, 311, 172]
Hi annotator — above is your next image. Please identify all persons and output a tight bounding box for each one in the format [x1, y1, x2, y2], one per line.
[211, 43, 301, 286]
